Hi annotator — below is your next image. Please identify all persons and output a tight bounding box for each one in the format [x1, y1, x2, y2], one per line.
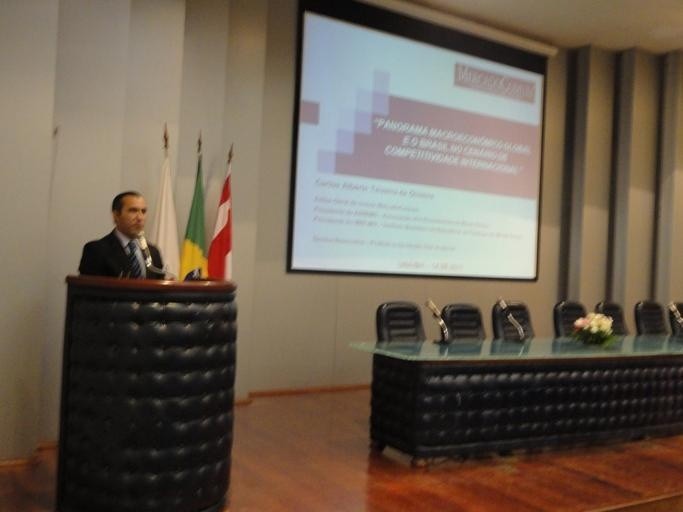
[77, 191, 175, 280]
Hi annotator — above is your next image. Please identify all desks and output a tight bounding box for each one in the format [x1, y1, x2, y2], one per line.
[357, 333, 682, 461]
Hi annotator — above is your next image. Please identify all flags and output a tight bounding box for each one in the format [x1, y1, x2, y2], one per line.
[178, 152, 209, 280]
[204, 158, 231, 282]
[146, 151, 180, 282]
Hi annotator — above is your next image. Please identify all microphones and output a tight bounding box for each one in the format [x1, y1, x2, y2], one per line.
[138, 230, 152, 265]
[497, 296, 511, 316]
[667, 302, 683, 324]
[424, 298, 441, 317]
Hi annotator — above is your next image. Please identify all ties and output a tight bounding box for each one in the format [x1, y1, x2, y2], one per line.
[128, 241, 141, 277]
